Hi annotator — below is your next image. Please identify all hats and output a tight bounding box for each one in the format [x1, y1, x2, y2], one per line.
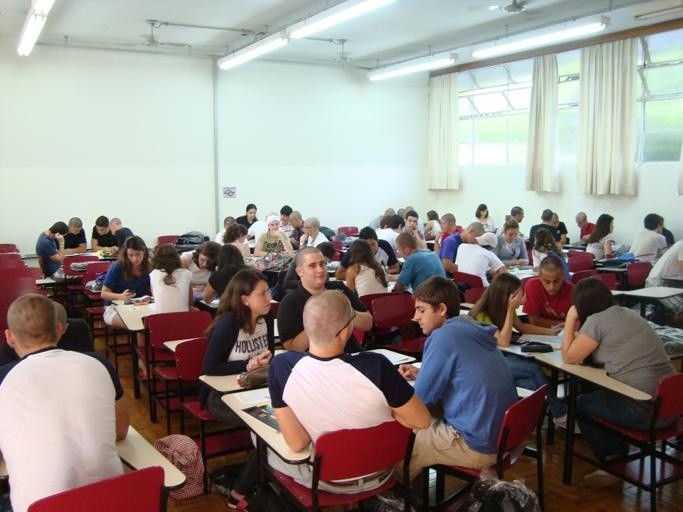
[475, 232, 498, 248]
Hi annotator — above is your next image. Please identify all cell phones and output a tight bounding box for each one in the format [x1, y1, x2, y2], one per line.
[127, 289, 135, 294]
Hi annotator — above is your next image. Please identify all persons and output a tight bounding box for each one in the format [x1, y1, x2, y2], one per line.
[439, 222, 485, 273]
[376, 215, 406, 252]
[396, 276, 522, 482]
[0, 293, 130, 512]
[251, 290, 432, 495]
[575, 212, 596, 241]
[472, 203, 495, 230]
[455, 232, 508, 287]
[562, 278, 681, 487]
[53, 301, 70, 344]
[586, 214, 615, 259]
[278, 247, 373, 352]
[279, 205, 294, 236]
[424, 210, 442, 239]
[289, 211, 305, 250]
[393, 233, 448, 292]
[101, 236, 152, 377]
[255, 213, 294, 256]
[629, 214, 667, 263]
[36, 221, 69, 280]
[532, 229, 571, 278]
[529, 208, 557, 241]
[205, 244, 253, 304]
[552, 212, 568, 244]
[223, 224, 249, 259]
[497, 206, 524, 236]
[434, 213, 464, 255]
[521, 256, 576, 326]
[346, 239, 389, 298]
[375, 215, 392, 231]
[467, 272, 580, 433]
[401, 210, 425, 242]
[149, 244, 193, 313]
[643, 239, 683, 325]
[92, 216, 113, 252]
[110, 217, 134, 252]
[64, 217, 87, 254]
[215, 216, 236, 245]
[657, 216, 675, 244]
[491, 220, 529, 266]
[300, 216, 329, 249]
[335, 226, 401, 280]
[180, 242, 221, 293]
[236, 204, 259, 228]
[199, 269, 276, 508]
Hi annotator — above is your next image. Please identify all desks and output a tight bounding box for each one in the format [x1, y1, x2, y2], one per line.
[113, 303, 154, 399]
[199, 348, 416, 393]
[611, 286, 683, 319]
[498, 343, 568, 446]
[221, 363, 548, 487]
[596, 267, 628, 272]
[460, 302, 529, 316]
[36, 278, 56, 285]
[0, 423, 187, 489]
[535, 351, 651, 486]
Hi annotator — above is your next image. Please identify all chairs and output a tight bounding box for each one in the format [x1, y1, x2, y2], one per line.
[338, 227, 358, 237]
[562, 372, 683, 511]
[150, 311, 214, 436]
[158, 236, 178, 244]
[371, 295, 416, 329]
[63, 256, 99, 312]
[104, 326, 133, 373]
[135, 315, 176, 421]
[629, 261, 652, 289]
[264, 421, 416, 512]
[0, 243, 49, 345]
[567, 252, 593, 272]
[464, 287, 487, 302]
[591, 274, 615, 290]
[360, 294, 402, 315]
[28, 466, 168, 512]
[571, 272, 595, 284]
[422, 384, 550, 512]
[84, 262, 111, 348]
[452, 272, 483, 290]
[176, 337, 255, 496]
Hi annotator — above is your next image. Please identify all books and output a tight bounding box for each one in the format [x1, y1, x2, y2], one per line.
[240, 404, 279, 432]
[517, 332, 562, 349]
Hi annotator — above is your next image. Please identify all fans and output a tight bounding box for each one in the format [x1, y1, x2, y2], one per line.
[316, 39, 363, 70]
[119, 20, 186, 48]
[468, 0, 544, 17]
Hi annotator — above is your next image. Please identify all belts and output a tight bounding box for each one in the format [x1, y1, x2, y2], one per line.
[325, 464, 395, 487]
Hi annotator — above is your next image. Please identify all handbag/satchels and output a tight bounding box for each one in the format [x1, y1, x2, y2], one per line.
[176, 231, 204, 245]
[154, 433, 204, 501]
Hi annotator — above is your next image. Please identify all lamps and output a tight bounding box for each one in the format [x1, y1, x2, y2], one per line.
[367, 53, 458, 83]
[17, 0, 57, 57]
[473, 16, 611, 62]
[217, 0, 397, 71]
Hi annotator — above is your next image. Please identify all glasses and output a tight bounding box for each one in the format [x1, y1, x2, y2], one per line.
[305, 226, 312, 228]
[335, 311, 357, 339]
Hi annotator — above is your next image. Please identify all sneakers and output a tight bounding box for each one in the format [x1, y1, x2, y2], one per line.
[554, 414, 581, 433]
[584, 463, 626, 487]
[226, 491, 240, 509]
